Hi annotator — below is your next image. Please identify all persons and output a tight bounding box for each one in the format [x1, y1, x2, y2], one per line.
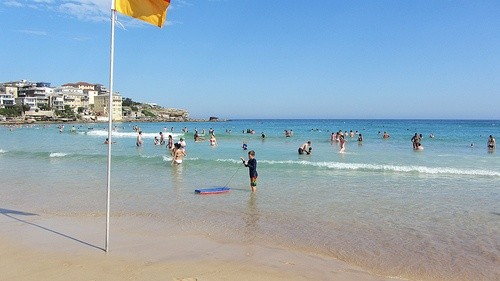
[226, 128, 232, 134]
[194, 127, 216, 147]
[58, 125, 93, 132]
[298, 141, 312, 155]
[284, 129, 293, 137]
[378, 130, 389, 138]
[488, 135, 496, 150]
[240, 150, 258, 191]
[242, 128, 266, 139]
[241, 141, 247, 150]
[133, 125, 144, 147]
[3, 124, 51, 132]
[331, 130, 363, 152]
[154, 127, 188, 165]
[104, 125, 124, 144]
[311, 128, 330, 132]
[411, 133, 434, 150]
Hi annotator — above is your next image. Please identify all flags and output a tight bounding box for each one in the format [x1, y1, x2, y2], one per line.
[114, 0, 171, 28]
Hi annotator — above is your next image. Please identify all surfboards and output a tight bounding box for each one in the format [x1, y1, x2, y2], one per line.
[195, 187, 230, 194]
